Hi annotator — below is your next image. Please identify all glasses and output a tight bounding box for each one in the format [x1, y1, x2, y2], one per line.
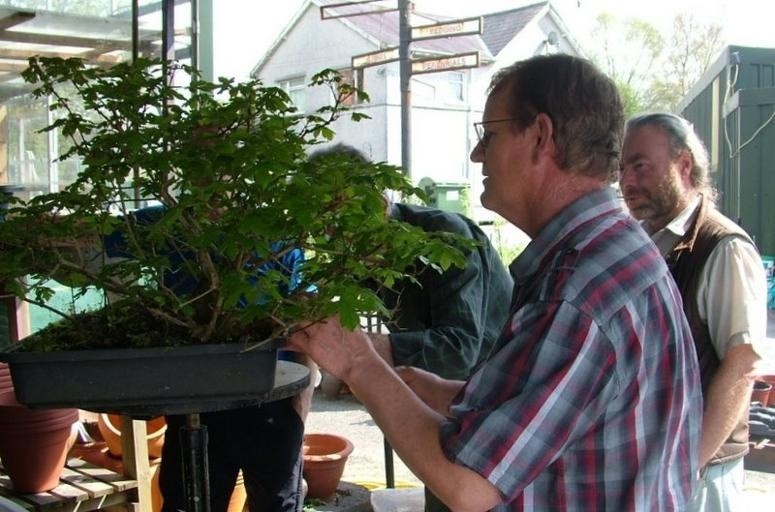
[473, 118, 532, 147]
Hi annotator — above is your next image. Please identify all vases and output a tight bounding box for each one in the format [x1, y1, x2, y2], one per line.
[752, 375, 774, 409]
[302, 433, 354, 498]
[751, 380, 773, 407]
[1, 358, 249, 512]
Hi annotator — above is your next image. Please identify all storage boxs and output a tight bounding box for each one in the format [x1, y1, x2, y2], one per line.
[766, 277, 775, 310]
[761, 256, 775, 278]
[371, 487, 427, 512]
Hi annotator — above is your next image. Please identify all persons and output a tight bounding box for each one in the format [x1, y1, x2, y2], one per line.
[45, 111, 325, 511]
[274, 53, 706, 512]
[293, 144, 524, 512]
[617, 113, 767, 512]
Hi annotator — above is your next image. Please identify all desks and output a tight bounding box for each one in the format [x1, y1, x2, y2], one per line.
[84, 359, 310, 512]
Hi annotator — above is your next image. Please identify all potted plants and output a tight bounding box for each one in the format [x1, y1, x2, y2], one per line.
[1, 47, 491, 408]
